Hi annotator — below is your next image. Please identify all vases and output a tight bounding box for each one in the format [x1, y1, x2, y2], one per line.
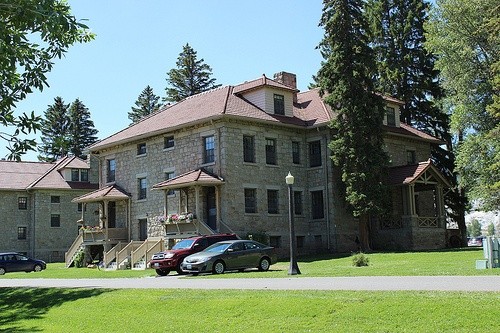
[160, 221, 164, 223]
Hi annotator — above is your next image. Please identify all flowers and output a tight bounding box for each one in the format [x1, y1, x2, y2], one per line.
[172, 213, 193, 220]
[159, 216, 166, 221]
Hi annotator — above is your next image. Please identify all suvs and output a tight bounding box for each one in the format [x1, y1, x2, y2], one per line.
[467, 237, 483, 247]
[150, 232, 239, 277]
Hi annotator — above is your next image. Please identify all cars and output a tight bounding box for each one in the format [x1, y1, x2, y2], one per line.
[0, 252, 46, 276]
[180, 239, 278, 278]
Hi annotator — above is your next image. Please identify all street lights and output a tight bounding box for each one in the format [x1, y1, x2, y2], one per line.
[285, 170, 301, 276]
